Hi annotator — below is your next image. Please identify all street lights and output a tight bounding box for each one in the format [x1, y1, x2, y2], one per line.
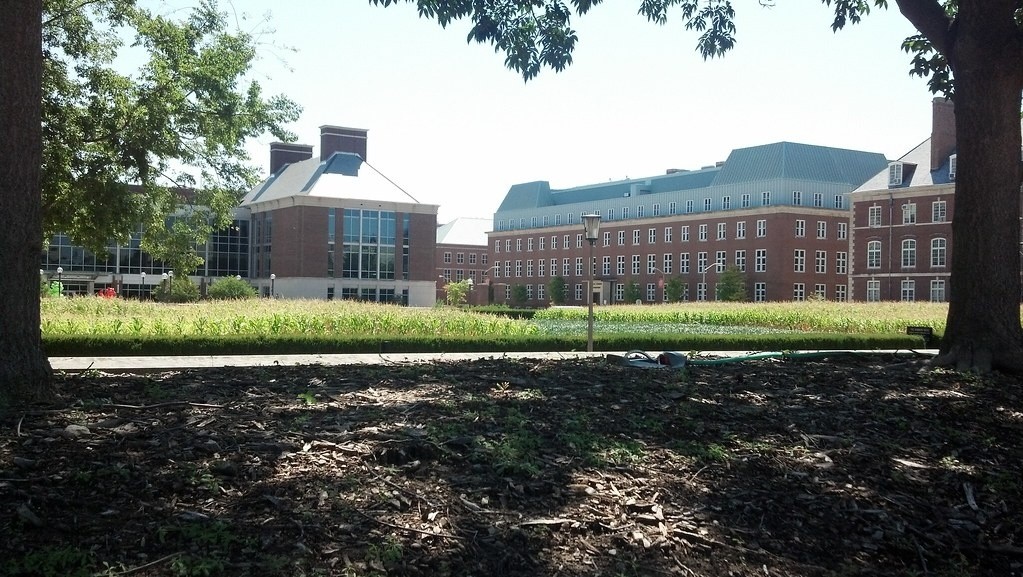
[468, 278, 473, 309]
[162, 273, 168, 292]
[702, 263, 722, 301]
[581, 214, 601, 352]
[168, 271, 173, 294]
[438, 275, 449, 306]
[270, 273, 276, 297]
[57, 267, 63, 299]
[141, 272, 146, 298]
